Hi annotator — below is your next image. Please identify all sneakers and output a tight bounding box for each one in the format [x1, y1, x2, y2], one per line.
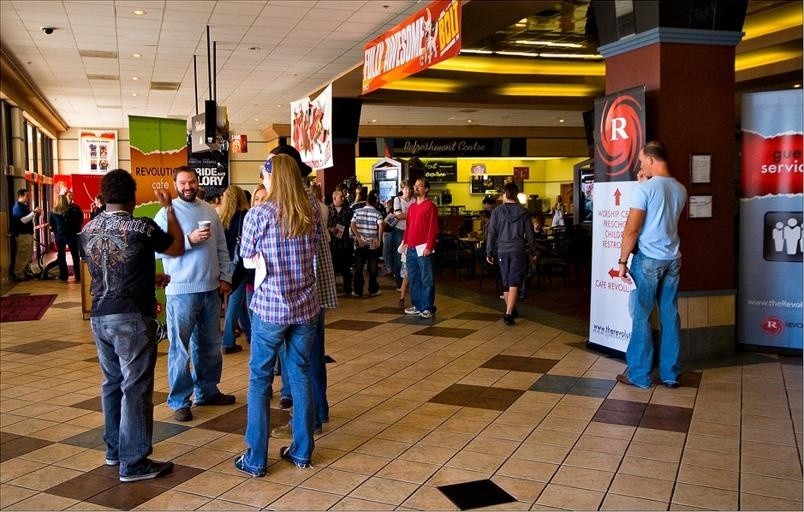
[503, 308, 517, 326]
[173, 408, 192, 421]
[404, 306, 421, 314]
[14, 275, 33, 281]
[352, 289, 382, 297]
[397, 287, 406, 307]
[381, 272, 393, 276]
[269, 423, 292, 437]
[106, 458, 173, 483]
[279, 400, 292, 408]
[223, 329, 244, 354]
[234, 457, 267, 476]
[280, 446, 310, 469]
[193, 392, 237, 405]
[419, 309, 435, 318]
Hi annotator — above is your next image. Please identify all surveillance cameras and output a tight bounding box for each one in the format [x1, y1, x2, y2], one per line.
[41, 26, 56, 34]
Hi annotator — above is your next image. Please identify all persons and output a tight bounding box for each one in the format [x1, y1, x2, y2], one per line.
[328, 176, 438, 318]
[233, 154, 321, 478]
[151, 166, 235, 422]
[198, 184, 330, 355]
[483, 182, 566, 326]
[8, 190, 110, 285]
[79, 169, 184, 483]
[617, 139, 689, 388]
[269, 145, 338, 438]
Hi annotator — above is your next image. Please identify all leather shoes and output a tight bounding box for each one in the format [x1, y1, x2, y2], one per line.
[616, 374, 635, 385]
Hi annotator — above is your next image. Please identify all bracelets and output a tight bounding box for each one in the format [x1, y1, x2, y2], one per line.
[166, 204, 174, 212]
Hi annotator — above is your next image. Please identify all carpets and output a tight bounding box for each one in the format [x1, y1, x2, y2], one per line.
[0, 294, 59, 322]
[442, 253, 590, 324]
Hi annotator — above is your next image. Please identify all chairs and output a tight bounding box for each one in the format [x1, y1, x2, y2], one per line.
[442, 229, 581, 293]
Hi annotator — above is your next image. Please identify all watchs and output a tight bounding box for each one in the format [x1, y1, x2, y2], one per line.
[618, 258, 627, 264]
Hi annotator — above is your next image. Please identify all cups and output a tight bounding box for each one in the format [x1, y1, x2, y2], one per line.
[197, 220, 211, 239]
